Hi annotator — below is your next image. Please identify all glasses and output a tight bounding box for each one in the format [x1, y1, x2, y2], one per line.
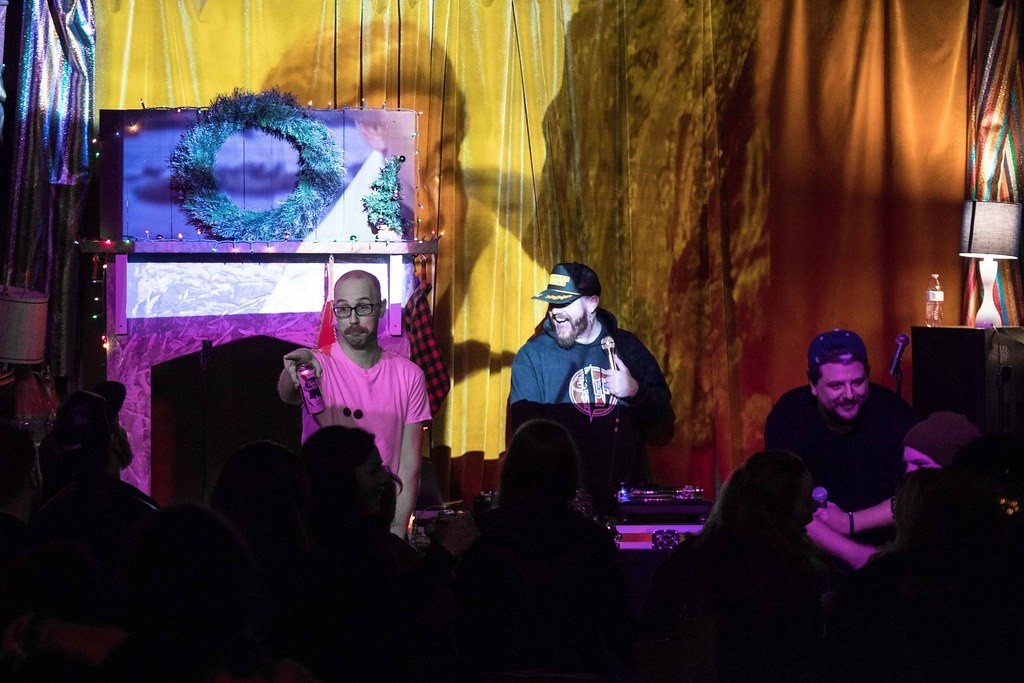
[333, 302, 383, 318]
[390, 472, 403, 497]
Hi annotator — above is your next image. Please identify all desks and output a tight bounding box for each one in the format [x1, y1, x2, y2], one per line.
[911, 326, 1024, 472]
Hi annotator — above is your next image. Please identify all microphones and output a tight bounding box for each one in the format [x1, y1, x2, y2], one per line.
[811, 486, 829, 509]
[601, 336, 618, 370]
[889, 334, 910, 376]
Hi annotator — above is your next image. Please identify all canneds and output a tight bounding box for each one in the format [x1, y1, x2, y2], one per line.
[295, 362, 326, 416]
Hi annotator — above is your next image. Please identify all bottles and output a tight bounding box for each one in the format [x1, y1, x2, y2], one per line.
[925, 273, 945, 327]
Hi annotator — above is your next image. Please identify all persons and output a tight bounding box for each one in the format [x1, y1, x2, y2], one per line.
[453, 420, 639, 676]
[510, 262, 676, 511]
[277, 270, 433, 541]
[805, 410, 980, 568]
[260, 22, 552, 459]
[0, 381, 447, 683]
[667, 433, 1024, 683]
[764, 328, 913, 511]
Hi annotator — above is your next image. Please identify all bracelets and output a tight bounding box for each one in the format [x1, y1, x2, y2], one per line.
[847, 510, 855, 537]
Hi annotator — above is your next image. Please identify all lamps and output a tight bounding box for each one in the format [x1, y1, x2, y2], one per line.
[958, 199, 1022, 328]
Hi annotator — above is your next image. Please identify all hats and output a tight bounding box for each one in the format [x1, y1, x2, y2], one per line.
[532, 262, 601, 306]
[808, 330, 869, 367]
[903, 410, 981, 463]
